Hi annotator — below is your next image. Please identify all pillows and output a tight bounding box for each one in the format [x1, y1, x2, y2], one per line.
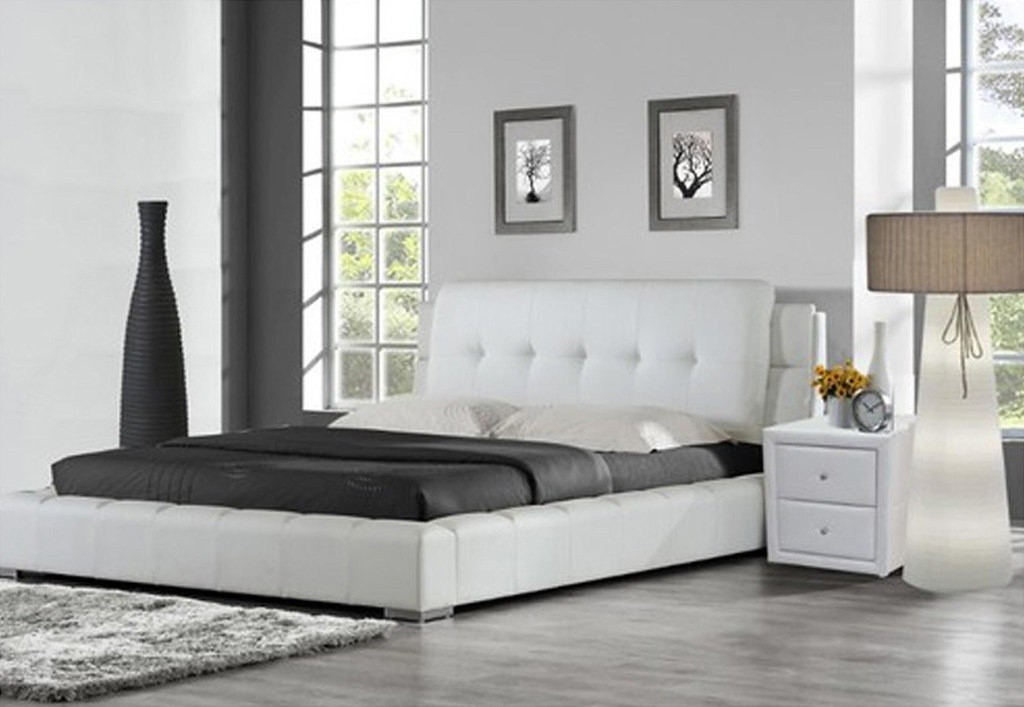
[328, 392, 522, 442]
[482, 401, 742, 455]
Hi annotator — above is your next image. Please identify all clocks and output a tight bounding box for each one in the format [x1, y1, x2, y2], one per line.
[849, 387, 894, 433]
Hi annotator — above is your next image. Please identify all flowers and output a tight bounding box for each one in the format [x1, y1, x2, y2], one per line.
[809, 362, 867, 397]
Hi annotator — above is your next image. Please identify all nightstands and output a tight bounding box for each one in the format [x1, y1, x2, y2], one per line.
[761, 411, 917, 578]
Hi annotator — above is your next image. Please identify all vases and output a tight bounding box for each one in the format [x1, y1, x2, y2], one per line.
[825, 392, 859, 431]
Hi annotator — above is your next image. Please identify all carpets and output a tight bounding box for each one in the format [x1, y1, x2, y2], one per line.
[0, 575, 399, 706]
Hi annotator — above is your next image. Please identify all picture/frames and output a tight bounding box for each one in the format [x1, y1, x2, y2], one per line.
[493, 103, 580, 234]
[645, 92, 741, 233]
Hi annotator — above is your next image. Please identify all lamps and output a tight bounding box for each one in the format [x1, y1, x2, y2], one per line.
[863, 207, 1023, 391]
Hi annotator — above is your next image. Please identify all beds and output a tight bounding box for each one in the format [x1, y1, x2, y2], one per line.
[0, 274, 831, 626]
